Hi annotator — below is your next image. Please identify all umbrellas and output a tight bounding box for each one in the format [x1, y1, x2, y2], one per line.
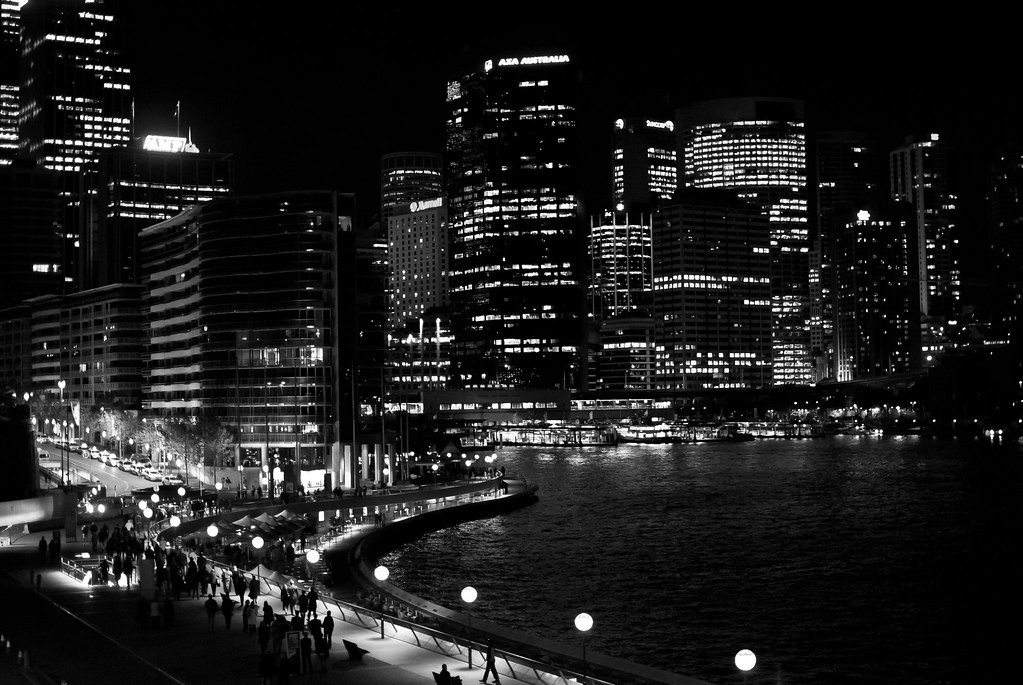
[187, 509, 312, 562]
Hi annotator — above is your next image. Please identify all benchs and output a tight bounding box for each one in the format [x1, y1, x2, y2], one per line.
[324, 493, 339, 500]
[396, 486, 413, 493]
[220, 593, 239, 607]
[369, 489, 389, 496]
[343, 639, 370, 660]
[433, 672, 462, 685]
[243, 501, 264, 510]
[274, 614, 291, 627]
[464, 479, 473, 484]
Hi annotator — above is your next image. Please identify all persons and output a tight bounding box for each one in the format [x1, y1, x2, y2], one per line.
[81, 523, 334, 685]
[123, 476, 262, 533]
[440, 664, 462, 681]
[38, 536, 57, 562]
[375, 513, 386, 528]
[274, 479, 375, 499]
[44, 471, 51, 483]
[479, 639, 500, 684]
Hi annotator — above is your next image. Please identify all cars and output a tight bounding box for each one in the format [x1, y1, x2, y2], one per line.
[161, 475, 183, 487]
[33, 429, 163, 482]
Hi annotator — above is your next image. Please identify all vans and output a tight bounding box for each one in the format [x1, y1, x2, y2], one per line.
[718, 425, 737, 438]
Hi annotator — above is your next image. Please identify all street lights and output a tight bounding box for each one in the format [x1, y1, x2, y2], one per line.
[382, 466, 393, 495]
[170, 515, 182, 548]
[143, 507, 153, 544]
[374, 566, 390, 639]
[207, 525, 219, 570]
[432, 451, 499, 487]
[215, 481, 223, 514]
[251, 536, 264, 579]
[306, 550, 320, 592]
[177, 487, 186, 518]
[735, 648, 756, 685]
[461, 586, 478, 640]
[574, 612, 594, 675]
